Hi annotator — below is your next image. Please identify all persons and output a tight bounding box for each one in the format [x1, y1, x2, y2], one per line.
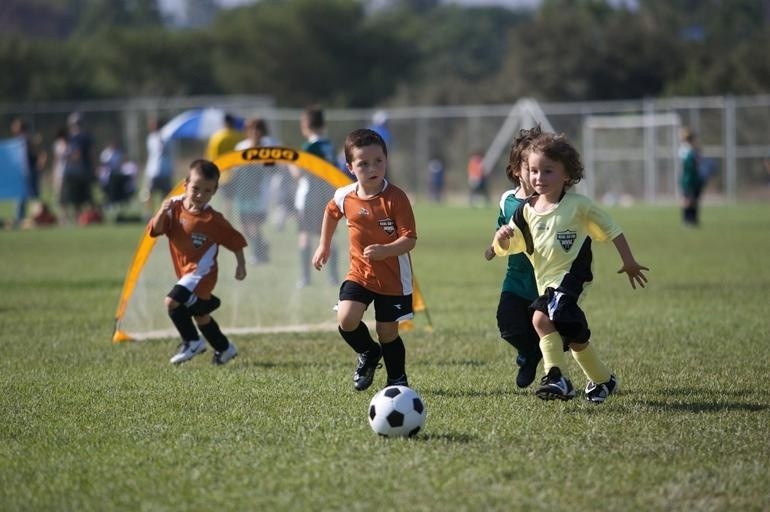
[286, 106, 341, 291]
[144, 112, 273, 264]
[428, 152, 447, 206]
[6, 110, 138, 224]
[490, 133, 649, 405]
[339, 108, 394, 183]
[467, 150, 484, 209]
[311, 129, 416, 391]
[148, 160, 247, 366]
[484, 124, 549, 388]
[678, 130, 700, 227]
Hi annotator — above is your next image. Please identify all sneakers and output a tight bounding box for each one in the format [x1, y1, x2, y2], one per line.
[352, 343, 382, 391]
[210, 345, 238, 366]
[536, 367, 574, 400]
[169, 339, 205, 364]
[584, 375, 617, 402]
[516, 355, 542, 388]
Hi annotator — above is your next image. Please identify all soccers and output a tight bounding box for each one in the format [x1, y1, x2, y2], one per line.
[368, 385, 427, 438]
